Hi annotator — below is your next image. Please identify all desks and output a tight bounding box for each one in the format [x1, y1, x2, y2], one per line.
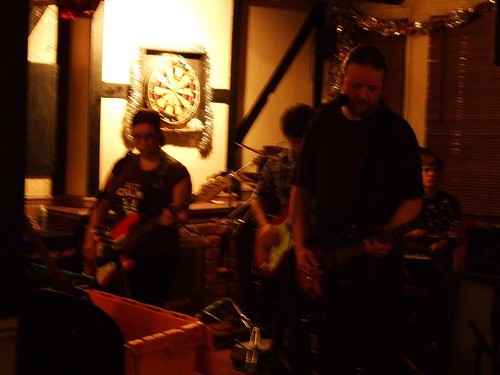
[46, 200, 249, 292]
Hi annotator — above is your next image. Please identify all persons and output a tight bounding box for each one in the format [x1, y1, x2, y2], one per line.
[84, 109, 193, 309]
[220, 43, 465, 375]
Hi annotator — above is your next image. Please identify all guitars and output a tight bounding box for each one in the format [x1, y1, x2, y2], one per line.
[254, 218, 294, 274]
[95, 193, 201, 286]
[295, 194, 457, 299]
